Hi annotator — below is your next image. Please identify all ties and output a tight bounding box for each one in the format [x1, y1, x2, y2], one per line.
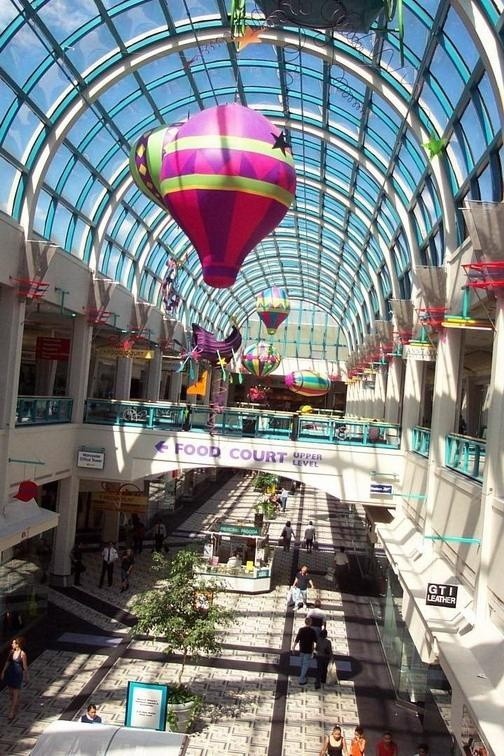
[108, 549, 110, 561]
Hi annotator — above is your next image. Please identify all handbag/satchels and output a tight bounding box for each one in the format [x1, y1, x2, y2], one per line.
[282, 531, 287, 536]
[319, 734, 330, 756]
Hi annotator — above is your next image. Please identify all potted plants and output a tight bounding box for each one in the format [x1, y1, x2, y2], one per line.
[126, 545, 249, 733]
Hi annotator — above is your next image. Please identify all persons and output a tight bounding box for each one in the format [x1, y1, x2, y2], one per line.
[122, 513, 167, 555]
[313, 630, 334, 689]
[319, 724, 348, 756]
[375, 729, 398, 756]
[303, 521, 316, 554]
[330, 546, 351, 591]
[0, 635, 28, 721]
[267, 479, 298, 512]
[348, 727, 366, 756]
[75, 703, 104, 756]
[95, 540, 118, 589]
[412, 743, 432, 756]
[462, 738, 473, 756]
[67, 538, 85, 588]
[119, 547, 135, 594]
[290, 566, 314, 612]
[305, 599, 326, 657]
[278, 520, 296, 552]
[36, 536, 51, 586]
[291, 618, 318, 686]
